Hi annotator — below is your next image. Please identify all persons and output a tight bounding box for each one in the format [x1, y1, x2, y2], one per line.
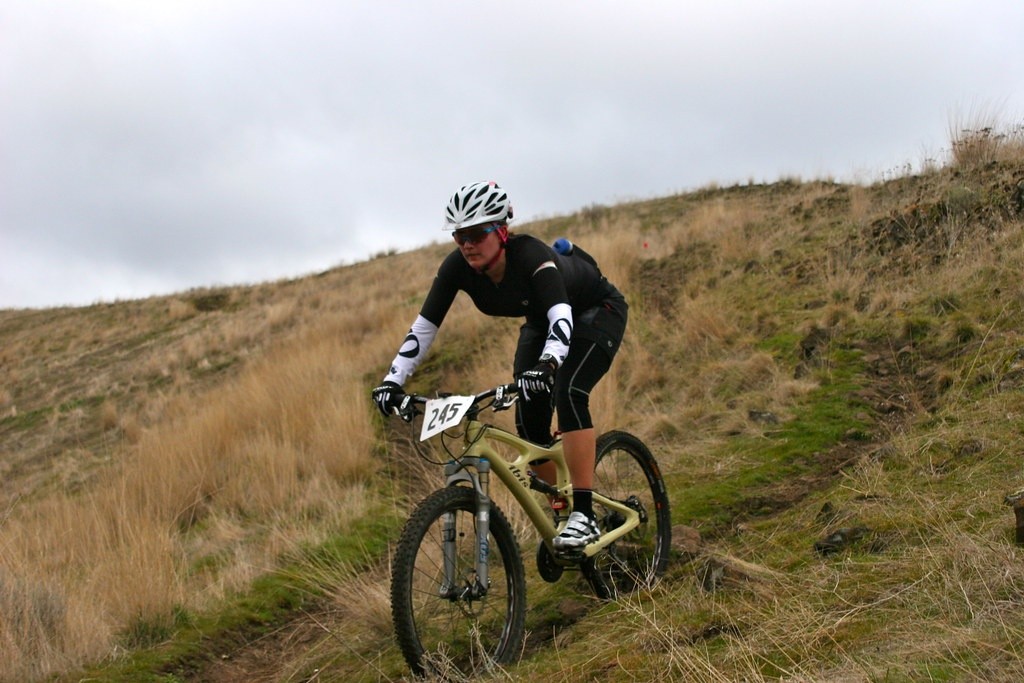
[372, 181, 630, 551]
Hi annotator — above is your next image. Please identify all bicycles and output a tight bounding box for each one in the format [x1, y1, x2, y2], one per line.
[378, 376, 672, 683]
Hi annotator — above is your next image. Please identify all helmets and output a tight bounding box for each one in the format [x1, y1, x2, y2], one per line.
[442, 181, 513, 230]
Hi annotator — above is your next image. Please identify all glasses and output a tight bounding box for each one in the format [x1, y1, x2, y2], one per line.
[452, 224, 500, 246]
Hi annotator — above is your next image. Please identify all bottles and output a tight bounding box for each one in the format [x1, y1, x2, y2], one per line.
[553, 238, 574, 255]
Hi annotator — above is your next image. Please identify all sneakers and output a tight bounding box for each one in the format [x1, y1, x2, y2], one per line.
[551, 510, 602, 551]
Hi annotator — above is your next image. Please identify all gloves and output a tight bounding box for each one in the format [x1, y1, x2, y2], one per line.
[372, 384, 411, 423]
[517, 362, 556, 403]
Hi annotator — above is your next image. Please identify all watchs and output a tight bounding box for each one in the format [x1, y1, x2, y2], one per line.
[537, 354, 558, 370]
